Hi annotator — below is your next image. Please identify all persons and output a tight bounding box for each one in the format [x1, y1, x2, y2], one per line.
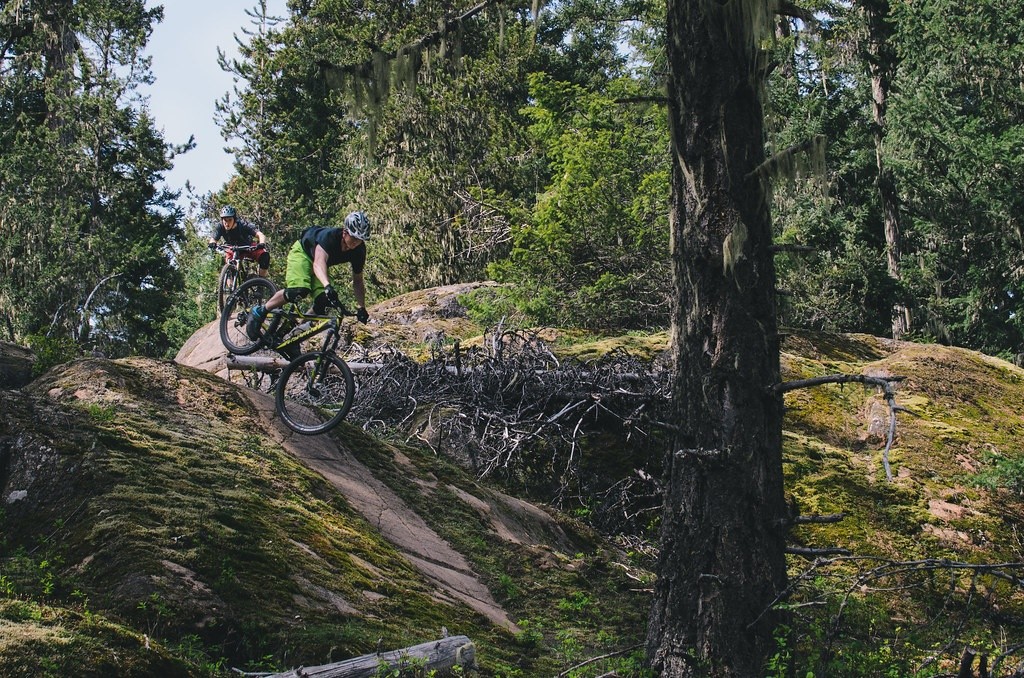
[208, 205, 269, 293]
[246, 211, 370, 341]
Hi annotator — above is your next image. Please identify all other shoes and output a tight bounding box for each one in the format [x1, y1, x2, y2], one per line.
[254, 287, 264, 299]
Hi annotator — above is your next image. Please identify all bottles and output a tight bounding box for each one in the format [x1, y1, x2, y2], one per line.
[280, 321, 317, 343]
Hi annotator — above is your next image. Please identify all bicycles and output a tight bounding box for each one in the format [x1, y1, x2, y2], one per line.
[207, 244, 262, 316]
[219, 276, 355, 436]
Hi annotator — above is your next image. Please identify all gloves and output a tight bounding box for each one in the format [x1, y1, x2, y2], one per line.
[208, 242, 217, 249]
[356, 307, 370, 325]
[254, 243, 266, 251]
[324, 284, 338, 301]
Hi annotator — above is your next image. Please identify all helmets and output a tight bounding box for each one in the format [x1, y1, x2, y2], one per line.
[345, 212, 373, 242]
[219, 205, 236, 217]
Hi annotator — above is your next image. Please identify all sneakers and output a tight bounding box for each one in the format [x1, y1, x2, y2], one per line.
[246, 306, 267, 342]
[285, 343, 305, 366]
